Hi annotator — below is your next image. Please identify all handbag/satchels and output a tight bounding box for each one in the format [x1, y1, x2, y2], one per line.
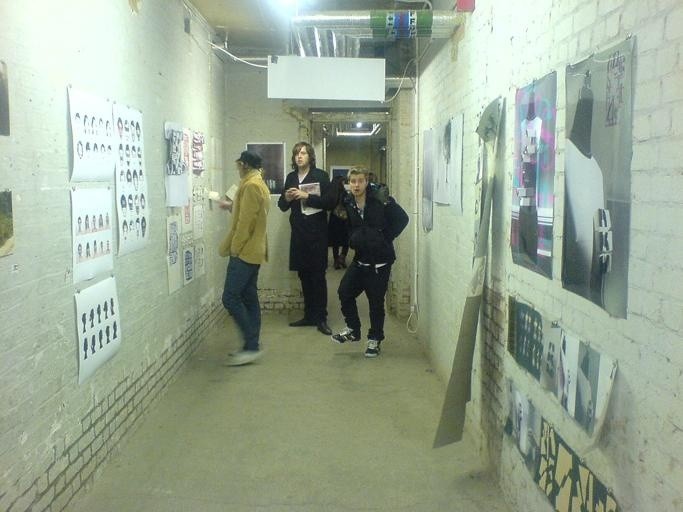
[333, 205, 348, 220]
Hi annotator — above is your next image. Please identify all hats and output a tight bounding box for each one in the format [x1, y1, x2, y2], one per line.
[238, 150, 263, 169]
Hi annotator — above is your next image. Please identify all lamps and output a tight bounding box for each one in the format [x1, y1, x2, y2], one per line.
[336, 122, 381, 136]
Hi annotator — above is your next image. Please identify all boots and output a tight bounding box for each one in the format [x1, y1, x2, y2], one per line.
[334, 253, 347, 269]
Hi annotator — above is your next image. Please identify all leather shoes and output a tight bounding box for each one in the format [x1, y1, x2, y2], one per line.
[317, 321, 332, 335]
[290, 319, 315, 326]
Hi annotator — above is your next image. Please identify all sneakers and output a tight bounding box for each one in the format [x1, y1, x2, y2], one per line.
[331, 327, 360, 342]
[226, 347, 262, 366]
[365, 339, 381, 357]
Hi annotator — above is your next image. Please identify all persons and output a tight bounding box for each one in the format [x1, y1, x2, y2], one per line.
[367, 172, 377, 186]
[329, 174, 351, 270]
[328, 165, 408, 358]
[216, 149, 271, 368]
[276, 141, 333, 336]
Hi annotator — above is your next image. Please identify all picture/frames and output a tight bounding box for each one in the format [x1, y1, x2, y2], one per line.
[246, 142, 286, 196]
[329, 165, 357, 191]
